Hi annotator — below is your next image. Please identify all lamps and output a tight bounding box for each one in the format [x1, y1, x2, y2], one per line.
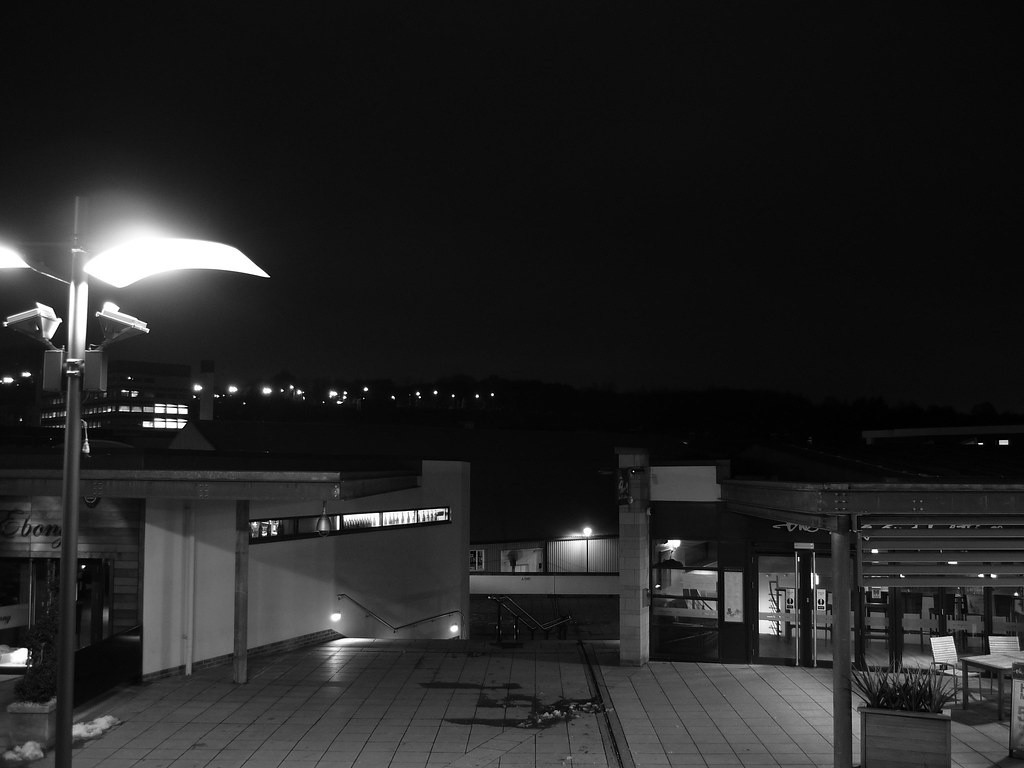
[317, 499, 332, 538]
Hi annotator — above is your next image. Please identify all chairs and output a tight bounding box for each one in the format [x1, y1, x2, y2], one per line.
[929, 635, 985, 706]
[988, 636, 1020, 695]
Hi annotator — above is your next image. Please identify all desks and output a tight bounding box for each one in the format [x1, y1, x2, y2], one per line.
[960, 650, 1024, 721]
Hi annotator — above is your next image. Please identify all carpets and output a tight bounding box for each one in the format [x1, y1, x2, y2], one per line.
[944, 692, 1012, 726]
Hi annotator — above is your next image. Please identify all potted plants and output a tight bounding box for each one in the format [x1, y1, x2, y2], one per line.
[6, 576, 59, 751]
[841, 658, 964, 768]
